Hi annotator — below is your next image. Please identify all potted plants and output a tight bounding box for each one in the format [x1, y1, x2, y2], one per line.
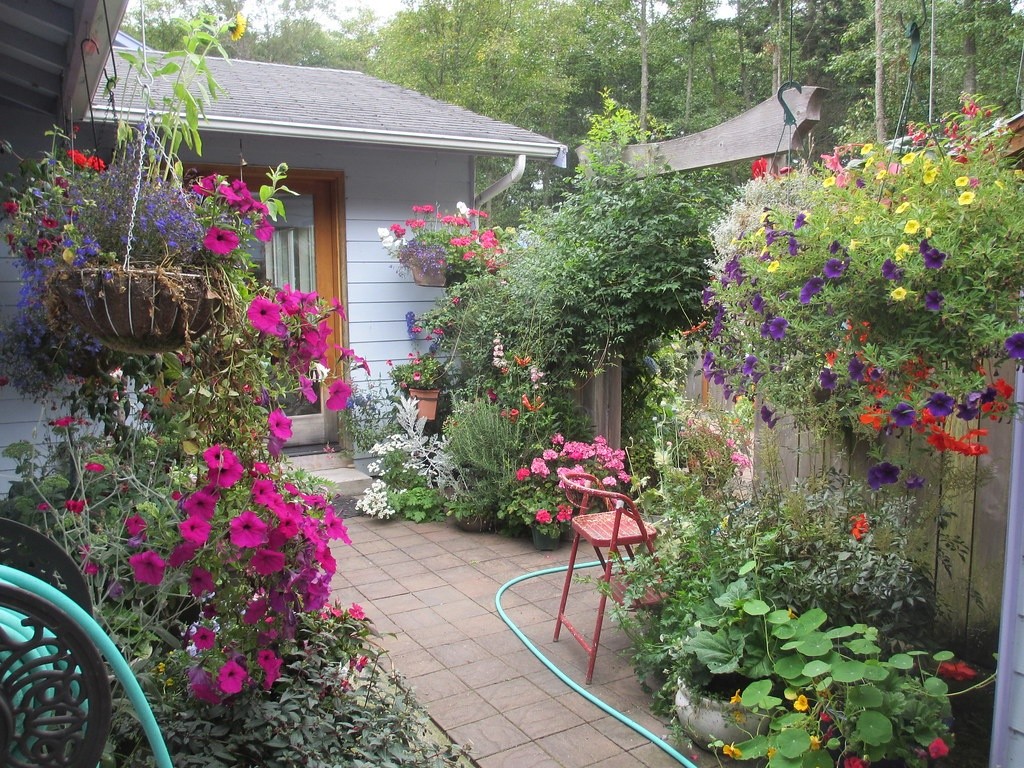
[444, 491, 490, 532]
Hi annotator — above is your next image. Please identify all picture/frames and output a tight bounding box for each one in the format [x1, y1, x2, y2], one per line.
[529, 524, 560, 551]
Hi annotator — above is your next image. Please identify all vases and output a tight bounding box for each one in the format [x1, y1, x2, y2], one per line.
[674, 675, 773, 755]
[407, 260, 448, 287]
[51, 268, 224, 356]
[408, 386, 441, 423]
[562, 515, 587, 543]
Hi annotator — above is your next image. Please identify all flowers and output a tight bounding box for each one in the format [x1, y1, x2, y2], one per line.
[677, 418, 753, 478]
[37, 414, 351, 705]
[656, 619, 778, 760]
[484, 329, 548, 425]
[240, 280, 371, 459]
[0, 8, 301, 355]
[385, 351, 448, 394]
[377, 199, 503, 278]
[698, 91, 1024, 490]
[707, 558, 998, 768]
[497, 469, 574, 539]
[530, 432, 631, 515]
